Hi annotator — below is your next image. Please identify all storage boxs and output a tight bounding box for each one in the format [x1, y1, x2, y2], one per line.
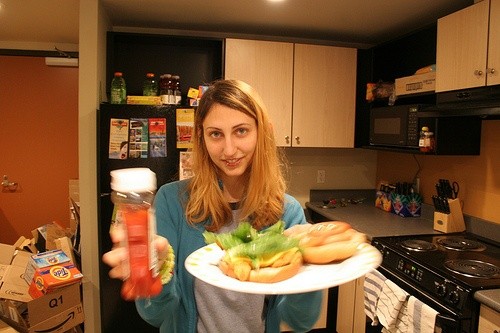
[0, 177, 82, 333]
[395, 71, 435, 96]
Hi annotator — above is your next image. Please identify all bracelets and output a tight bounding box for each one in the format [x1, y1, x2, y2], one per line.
[157, 243, 177, 286]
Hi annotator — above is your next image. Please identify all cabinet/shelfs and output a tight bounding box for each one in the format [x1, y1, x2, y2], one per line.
[220, 37, 358, 148]
[435, 1, 500, 92]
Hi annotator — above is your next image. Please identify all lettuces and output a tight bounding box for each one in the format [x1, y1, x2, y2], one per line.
[202, 219, 300, 262]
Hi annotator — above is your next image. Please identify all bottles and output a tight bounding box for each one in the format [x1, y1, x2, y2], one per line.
[110, 72, 127, 104]
[109, 166, 163, 303]
[143, 73, 182, 106]
[375, 182, 421, 217]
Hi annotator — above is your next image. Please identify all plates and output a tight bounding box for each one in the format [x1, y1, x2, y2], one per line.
[184, 242, 382, 296]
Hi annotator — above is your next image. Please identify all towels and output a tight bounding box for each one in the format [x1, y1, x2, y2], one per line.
[363, 268, 441, 333]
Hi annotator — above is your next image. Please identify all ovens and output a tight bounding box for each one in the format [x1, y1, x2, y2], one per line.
[365, 264, 478, 333]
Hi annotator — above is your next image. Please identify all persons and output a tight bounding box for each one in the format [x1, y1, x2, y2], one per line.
[101, 79, 323, 333]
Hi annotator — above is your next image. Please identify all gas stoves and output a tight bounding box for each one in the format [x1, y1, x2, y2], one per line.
[371, 230, 500, 318]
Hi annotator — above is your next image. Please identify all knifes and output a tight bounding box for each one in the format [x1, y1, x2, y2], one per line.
[431, 179, 454, 214]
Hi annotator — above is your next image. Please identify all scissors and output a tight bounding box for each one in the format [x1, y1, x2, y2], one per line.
[450, 181, 459, 199]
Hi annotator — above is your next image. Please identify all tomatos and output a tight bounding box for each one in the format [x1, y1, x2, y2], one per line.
[307, 221, 353, 236]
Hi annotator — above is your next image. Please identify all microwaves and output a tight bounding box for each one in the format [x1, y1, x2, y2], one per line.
[369, 103, 481, 156]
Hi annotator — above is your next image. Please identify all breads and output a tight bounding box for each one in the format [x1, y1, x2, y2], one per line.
[282, 221, 362, 264]
[220, 251, 305, 282]
